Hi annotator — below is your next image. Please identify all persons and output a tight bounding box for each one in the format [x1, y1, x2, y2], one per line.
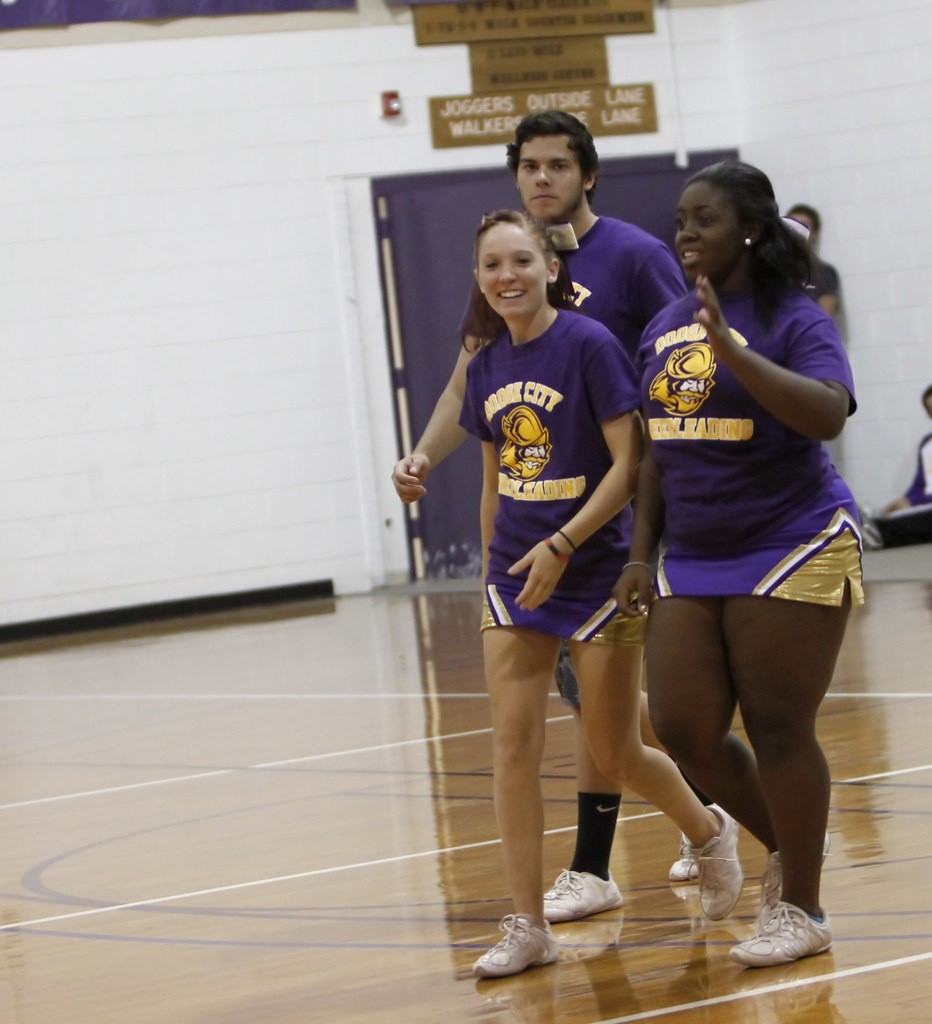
[458, 208, 746, 983]
[856, 386, 932, 550]
[785, 204, 838, 320]
[610, 156, 858, 965]
[390, 109, 701, 925]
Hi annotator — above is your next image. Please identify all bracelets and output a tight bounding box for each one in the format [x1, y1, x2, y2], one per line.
[622, 562, 654, 573]
[545, 536, 569, 562]
[558, 529, 580, 554]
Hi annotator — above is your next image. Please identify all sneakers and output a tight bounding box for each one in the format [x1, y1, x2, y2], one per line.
[544, 867, 624, 923]
[666, 831, 703, 881]
[728, 902, 833, 967]
[758, 829, 831, 908]
[686, 803, 744, 920]
[473, 913, 560, 977]
